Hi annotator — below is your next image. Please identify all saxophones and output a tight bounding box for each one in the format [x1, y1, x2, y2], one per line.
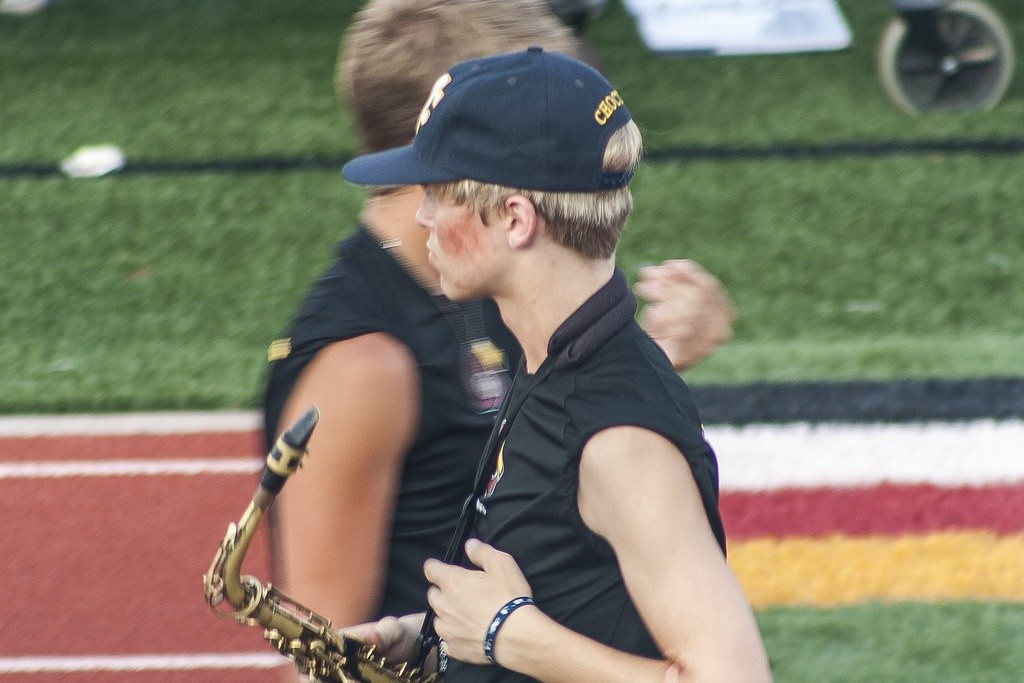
[202, 407, 437, 683]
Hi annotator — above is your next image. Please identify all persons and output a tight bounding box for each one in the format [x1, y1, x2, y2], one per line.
[338, 46, 772, 683]
[264, 0, 737, 683]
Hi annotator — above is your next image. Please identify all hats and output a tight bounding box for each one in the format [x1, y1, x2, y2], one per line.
[340, 45, 639, 194]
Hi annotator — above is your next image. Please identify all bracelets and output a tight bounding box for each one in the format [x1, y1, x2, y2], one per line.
[437, 637, 448, 675]
[484, 597, 535, 666]
